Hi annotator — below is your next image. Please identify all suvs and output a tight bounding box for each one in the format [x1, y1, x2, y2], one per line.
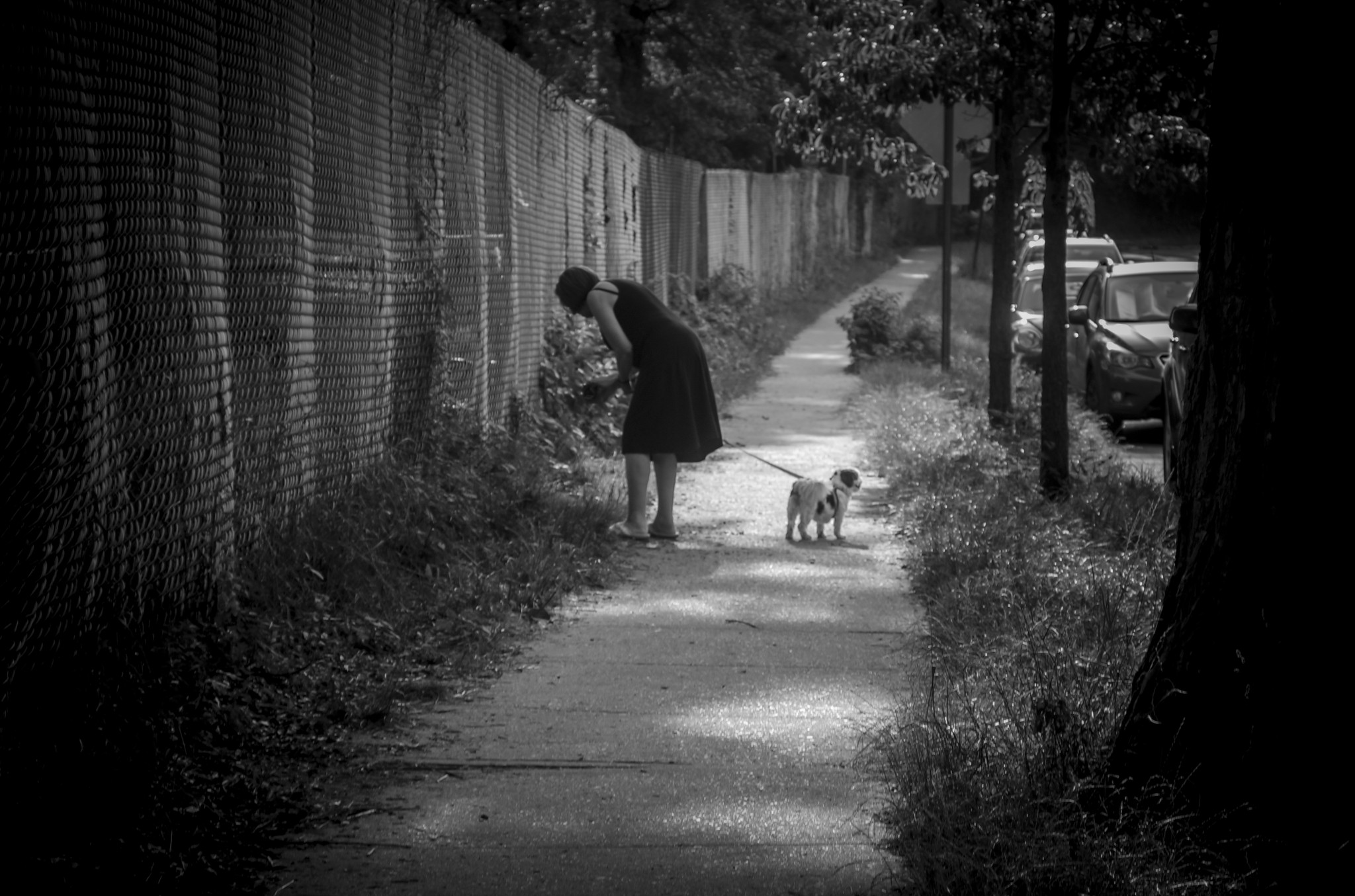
[1012, 227, 1128, 368]
[1067, 256, 1196, 435]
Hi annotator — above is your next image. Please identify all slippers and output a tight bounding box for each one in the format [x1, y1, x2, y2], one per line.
[648, 523, 679, 537]
[608, 522, 650, 538]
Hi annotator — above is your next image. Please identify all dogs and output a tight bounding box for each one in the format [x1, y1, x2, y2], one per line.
[783, 465, 864, 543]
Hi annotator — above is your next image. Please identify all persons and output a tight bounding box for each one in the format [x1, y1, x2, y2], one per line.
[554, 264, 724, 540]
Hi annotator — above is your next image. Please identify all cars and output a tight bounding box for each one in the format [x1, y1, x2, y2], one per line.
[1156, 279, 1199, 493]
